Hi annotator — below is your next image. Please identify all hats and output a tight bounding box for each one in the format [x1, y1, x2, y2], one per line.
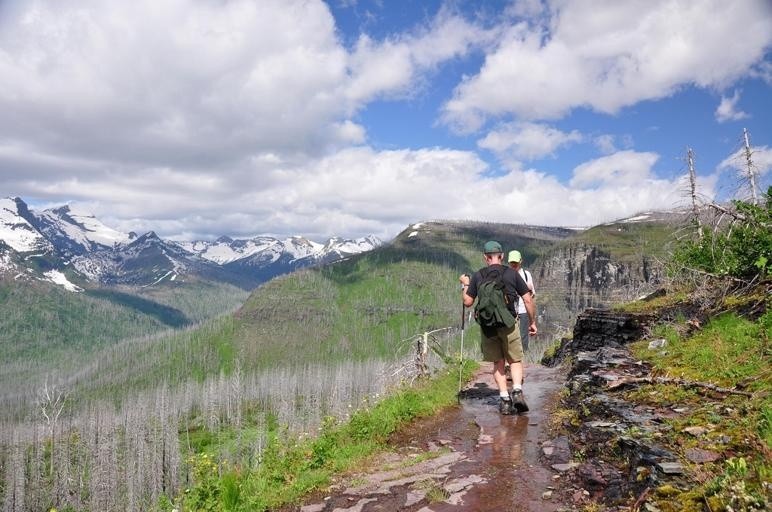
[507, 250, 521, 263]
[484, 241, 502, 253]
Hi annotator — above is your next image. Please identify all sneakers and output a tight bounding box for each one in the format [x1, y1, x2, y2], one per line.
[500, 396, 512, 415]
[512, 389, 530, 413]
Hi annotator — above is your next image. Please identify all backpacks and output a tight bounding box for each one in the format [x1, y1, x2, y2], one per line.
[474, 267, 515, 340]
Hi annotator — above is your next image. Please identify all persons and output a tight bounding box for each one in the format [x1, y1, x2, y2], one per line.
[459, 241, 538, 416]
